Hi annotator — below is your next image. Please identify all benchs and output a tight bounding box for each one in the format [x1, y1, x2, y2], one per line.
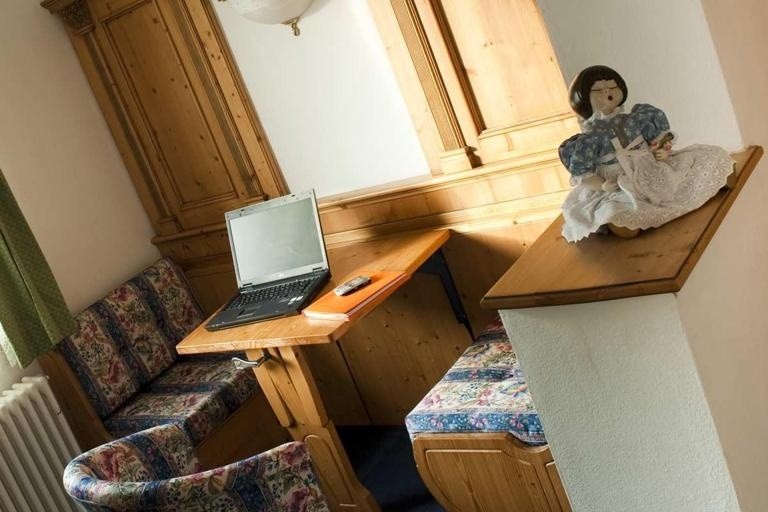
[401, 313, 572, 512]
[37, 259, 293, 466]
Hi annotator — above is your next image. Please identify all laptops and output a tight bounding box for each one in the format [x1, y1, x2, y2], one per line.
[203, 187, 332, 332]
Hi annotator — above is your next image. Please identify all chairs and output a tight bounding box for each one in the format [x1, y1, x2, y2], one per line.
[57, 423, 334, 512]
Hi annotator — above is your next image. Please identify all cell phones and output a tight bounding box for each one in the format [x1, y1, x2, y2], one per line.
[334, 274, 372, 297]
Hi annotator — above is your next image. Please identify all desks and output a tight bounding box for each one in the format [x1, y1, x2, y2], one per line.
[174, 223, 469, 512]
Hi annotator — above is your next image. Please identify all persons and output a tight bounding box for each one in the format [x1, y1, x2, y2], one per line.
[556, 63, 737, 244]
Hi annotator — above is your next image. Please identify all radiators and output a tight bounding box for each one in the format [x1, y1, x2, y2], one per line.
[0, 367, 96, 512]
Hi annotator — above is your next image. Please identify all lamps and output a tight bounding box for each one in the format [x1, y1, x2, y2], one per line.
[216, 0, 314, 38]
